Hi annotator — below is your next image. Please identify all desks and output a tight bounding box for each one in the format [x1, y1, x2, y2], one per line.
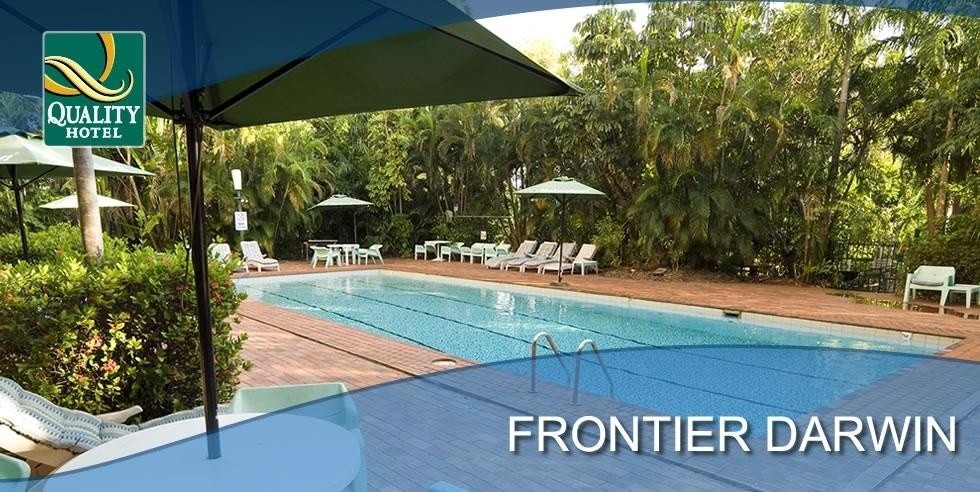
[941, 283, 980, 309]
[39, 409, 364, 492]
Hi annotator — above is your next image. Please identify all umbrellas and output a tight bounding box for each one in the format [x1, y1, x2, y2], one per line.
[306, 194, 376, 244]
[0, 134, 157, 261]
[0, 0, 588, 461]
[512, 171, 607, 280]
[39, 191, 130, 211]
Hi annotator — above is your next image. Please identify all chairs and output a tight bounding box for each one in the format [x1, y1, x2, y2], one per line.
[211, 235, 599, 275]
[230, 381, 366, 492]
[0, 344, 233, 491]
[900, 263, 957, 306]
